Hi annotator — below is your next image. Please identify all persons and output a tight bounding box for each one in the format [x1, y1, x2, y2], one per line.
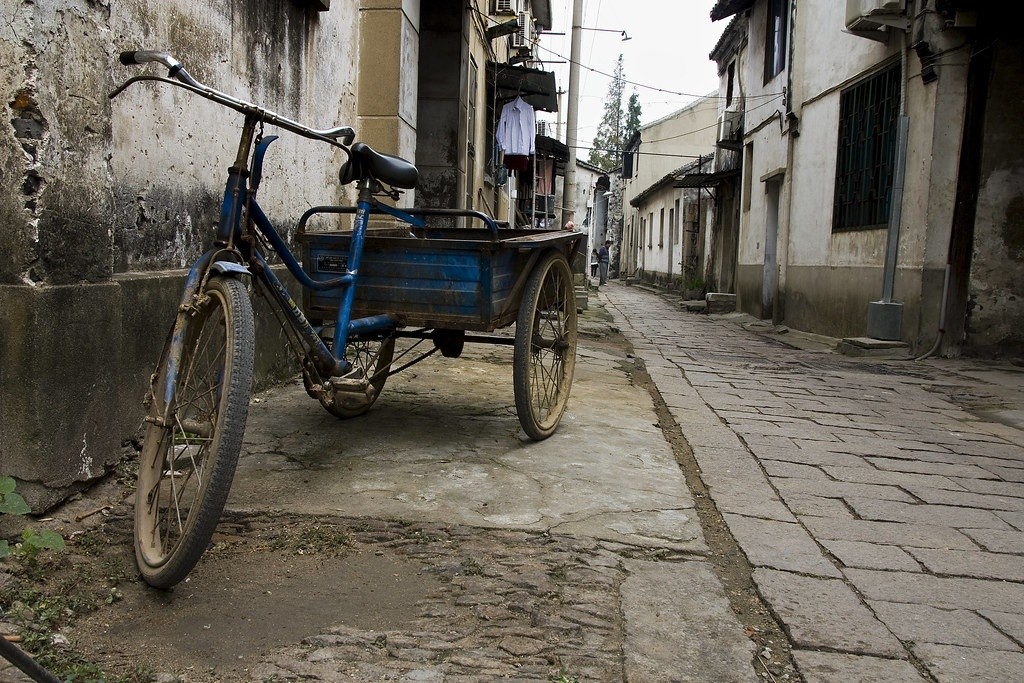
[598, 240, 612, 286]
[591, 249, 599, 279]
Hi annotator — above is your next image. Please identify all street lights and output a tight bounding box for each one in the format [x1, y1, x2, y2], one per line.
[562, 26, 632, 230]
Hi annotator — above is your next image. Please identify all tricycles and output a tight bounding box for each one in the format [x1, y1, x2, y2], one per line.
[108, 49, 586, 584]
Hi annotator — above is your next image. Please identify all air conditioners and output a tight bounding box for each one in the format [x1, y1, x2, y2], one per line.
[513, 11, 532, 50]
[496, 0, 519, 17]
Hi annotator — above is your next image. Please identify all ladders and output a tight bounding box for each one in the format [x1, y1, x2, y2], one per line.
[531, 150, 549, 229]
